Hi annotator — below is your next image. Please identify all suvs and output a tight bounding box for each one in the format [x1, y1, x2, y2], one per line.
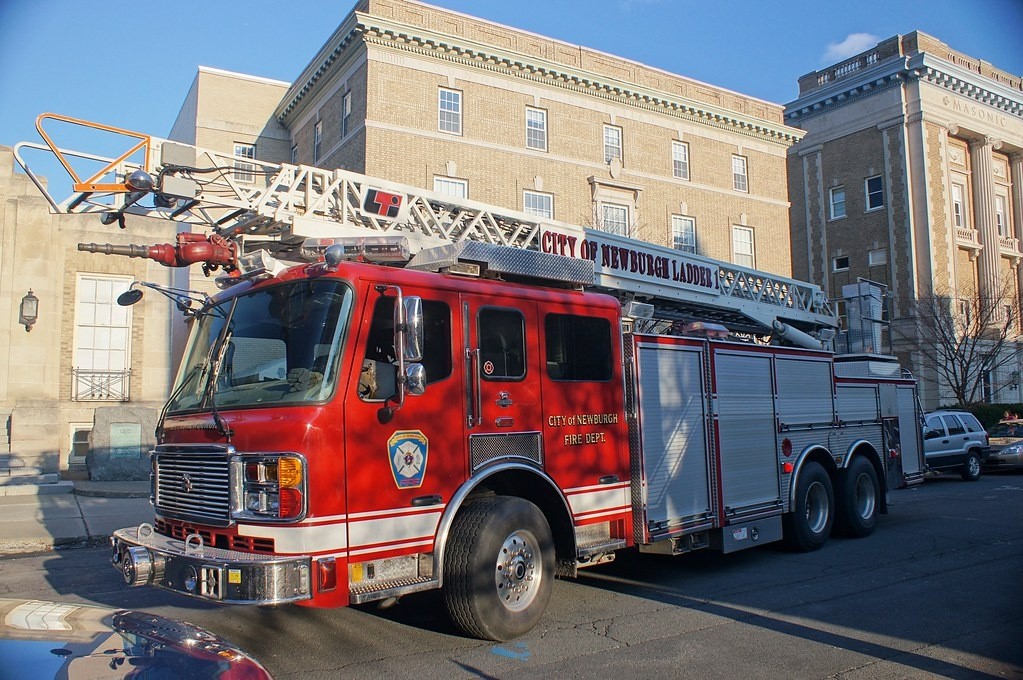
[919, 408, 991, 481]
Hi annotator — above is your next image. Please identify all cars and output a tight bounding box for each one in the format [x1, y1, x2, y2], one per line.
[1, 596, 274, 680]
[980, 419, 1023, 472]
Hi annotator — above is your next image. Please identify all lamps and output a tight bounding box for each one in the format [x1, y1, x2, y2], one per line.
[23, 288, 39, 332]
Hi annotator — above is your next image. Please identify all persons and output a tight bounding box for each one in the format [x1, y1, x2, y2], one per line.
[1000, 410, 1021, 434]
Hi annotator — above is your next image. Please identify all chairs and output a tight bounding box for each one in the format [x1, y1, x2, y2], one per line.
[313, 355, 398, 400]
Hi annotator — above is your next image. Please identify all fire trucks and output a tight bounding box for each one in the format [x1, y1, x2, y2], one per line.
[12, 112, 927, 645]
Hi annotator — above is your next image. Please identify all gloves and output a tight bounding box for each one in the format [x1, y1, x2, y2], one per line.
[287, 368, 323, 391]
